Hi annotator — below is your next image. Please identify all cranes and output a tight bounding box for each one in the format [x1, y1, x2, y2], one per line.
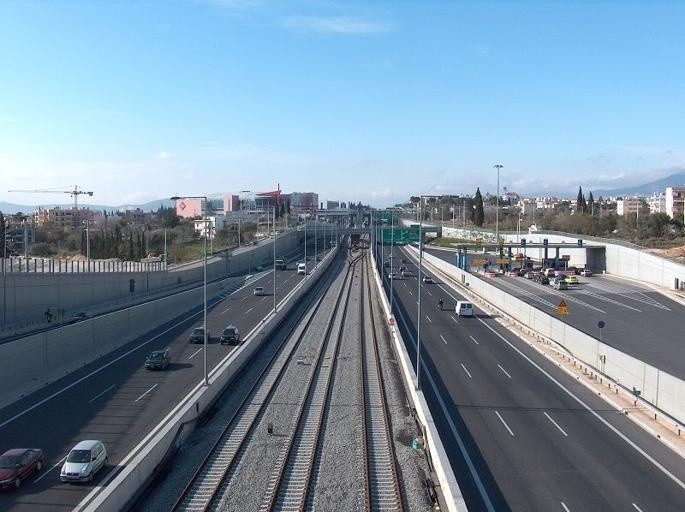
[7, 186, 94, 206]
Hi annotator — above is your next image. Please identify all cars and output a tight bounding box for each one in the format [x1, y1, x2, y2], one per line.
[537, 268, 577, 289]
[575, 267, 593, 276]
[60, 440, 108, 483]
[145, 350, 169, 369]
[276, 259, 286, 270]
[388, 272, 396, 279]
[423, 276, 432, 283]
[512, 267, 534, 279]
[189, 327, 210, 344]
[0, 448, 44, 491]
[220, 325, 239, 345]
[384, 252, 394, 266]
[253, 287, 264, 296]
[400, 259, 413, 277]
[298, 262, 306, 275]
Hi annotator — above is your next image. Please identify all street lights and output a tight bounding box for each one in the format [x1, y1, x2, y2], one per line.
[170, 196, 208, 385]
[494, 164, 502, 240]
[239, 190, 277, 313]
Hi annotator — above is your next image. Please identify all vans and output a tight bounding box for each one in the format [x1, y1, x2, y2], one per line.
[455, 300, 473, 317]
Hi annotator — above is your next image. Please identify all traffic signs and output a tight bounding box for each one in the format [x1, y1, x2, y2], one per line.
[372, 211, 421, 244]
